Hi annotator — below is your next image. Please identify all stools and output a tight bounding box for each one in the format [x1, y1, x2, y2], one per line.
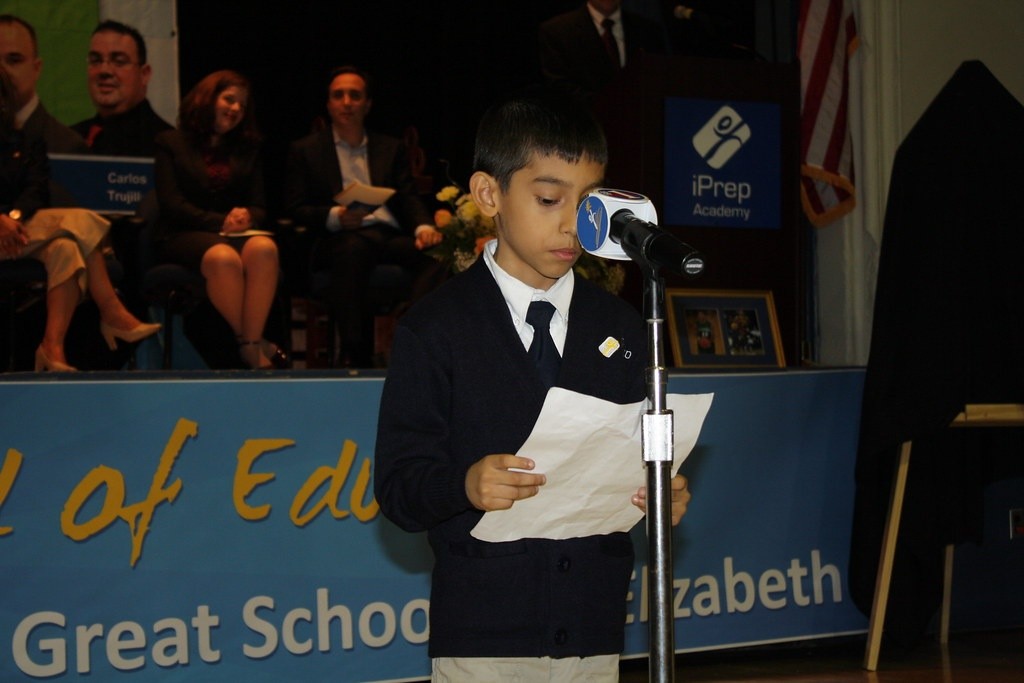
[0, 257, 346, 369]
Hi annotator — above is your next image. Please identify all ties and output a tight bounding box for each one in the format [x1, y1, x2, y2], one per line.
[525, 301, 561, 390]
[84, 124, 103, 148]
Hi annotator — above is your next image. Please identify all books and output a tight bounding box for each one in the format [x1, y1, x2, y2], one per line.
[333, 183, 395, 213]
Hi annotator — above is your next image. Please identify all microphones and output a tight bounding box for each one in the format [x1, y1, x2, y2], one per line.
[576, 187, 704, 274]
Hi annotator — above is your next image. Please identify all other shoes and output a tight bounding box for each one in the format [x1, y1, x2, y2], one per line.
[232, 331, 292, 378]
[349, 357, 375, 368]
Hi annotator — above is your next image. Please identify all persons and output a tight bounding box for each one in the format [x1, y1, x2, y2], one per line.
[694, 311, 716, 356]
[0, 71, 162, 372]
[374, 99, 692, 683]
[537, 0, 666, 105]
[726, 308, 760, 356]
[154, 68, 292, 370]
[0, 14, 92, 156]
[65, 18, 177, 158]
[290, 63, 442, 367]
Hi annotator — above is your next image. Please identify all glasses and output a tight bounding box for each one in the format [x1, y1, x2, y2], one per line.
[88, 53, 137, 68]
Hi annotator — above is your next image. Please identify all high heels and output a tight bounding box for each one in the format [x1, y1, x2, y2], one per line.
[34, 344, 77, 373]
[100, 319, 161, 351]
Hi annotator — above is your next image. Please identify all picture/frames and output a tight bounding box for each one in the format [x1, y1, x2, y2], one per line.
[663, 285, 785, 370]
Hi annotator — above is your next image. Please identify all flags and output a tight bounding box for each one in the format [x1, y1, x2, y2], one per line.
[795, 0, 857, 231]
